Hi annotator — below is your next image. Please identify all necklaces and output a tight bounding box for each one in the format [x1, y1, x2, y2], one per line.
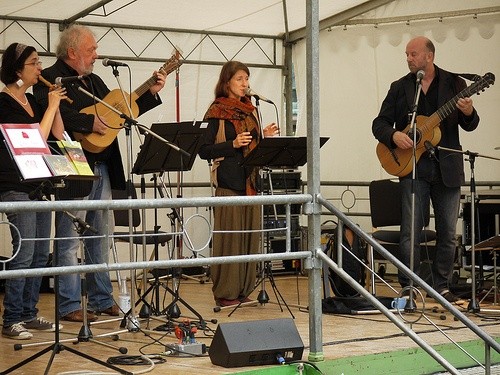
[6, 86, 28, 105]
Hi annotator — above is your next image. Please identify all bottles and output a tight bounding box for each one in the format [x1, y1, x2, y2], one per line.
[117, 276, 131, 318]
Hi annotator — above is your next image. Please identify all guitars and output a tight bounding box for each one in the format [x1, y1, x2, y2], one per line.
[376, 71, 496, 176]
[70, 54, 183, 155]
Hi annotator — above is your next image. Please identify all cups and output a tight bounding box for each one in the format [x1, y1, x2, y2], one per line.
[391, 298, 406, 309]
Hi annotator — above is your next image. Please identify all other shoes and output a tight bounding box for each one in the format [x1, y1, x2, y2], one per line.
[96, 304, 119, 316]
[59, 310, 98, 322]
[215, 294, 258, 307]
[402, 289, 417, 301]
[438, 288, 465, 305]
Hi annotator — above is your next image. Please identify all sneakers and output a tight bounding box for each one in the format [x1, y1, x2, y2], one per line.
[23, 317, 63, 333]
[2, 323, 33, 340]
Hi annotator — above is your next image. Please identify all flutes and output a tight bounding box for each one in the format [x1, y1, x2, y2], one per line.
[38, 75, 73, 104]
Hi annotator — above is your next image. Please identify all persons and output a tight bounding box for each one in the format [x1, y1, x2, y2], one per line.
[198, 61, 281, 308]
[32, 25, 167, 321]
[373, 36, 480, 313]
[0, 43, 68, 339]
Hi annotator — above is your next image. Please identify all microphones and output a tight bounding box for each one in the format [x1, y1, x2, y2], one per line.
[55, 75, 87, 85]
[29, 180, 48, 200]
[102, 58, 128, 67]
[244, 88, 274, 104]
[424, 140, 435, 155]
[416, 70, 425, 83]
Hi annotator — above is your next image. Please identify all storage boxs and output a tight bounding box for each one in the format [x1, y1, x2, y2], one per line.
[462, 190, 500, 272]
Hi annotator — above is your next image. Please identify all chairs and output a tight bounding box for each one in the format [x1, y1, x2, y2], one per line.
[111, 182, 172, 289]
[366, 179, 436, 296]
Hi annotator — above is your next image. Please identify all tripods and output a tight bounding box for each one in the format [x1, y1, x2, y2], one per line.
[214, 97, 330, 317]
[0, 67, 210, 375]
[350, 81, 500, 321]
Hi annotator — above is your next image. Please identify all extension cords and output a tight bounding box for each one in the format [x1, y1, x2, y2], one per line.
[164, 343, 206, 355]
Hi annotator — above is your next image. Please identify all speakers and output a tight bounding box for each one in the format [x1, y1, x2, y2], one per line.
[208, 318, 304, 368]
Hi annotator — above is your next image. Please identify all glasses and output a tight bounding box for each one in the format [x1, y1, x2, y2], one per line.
[24, 61, 42, 68]
